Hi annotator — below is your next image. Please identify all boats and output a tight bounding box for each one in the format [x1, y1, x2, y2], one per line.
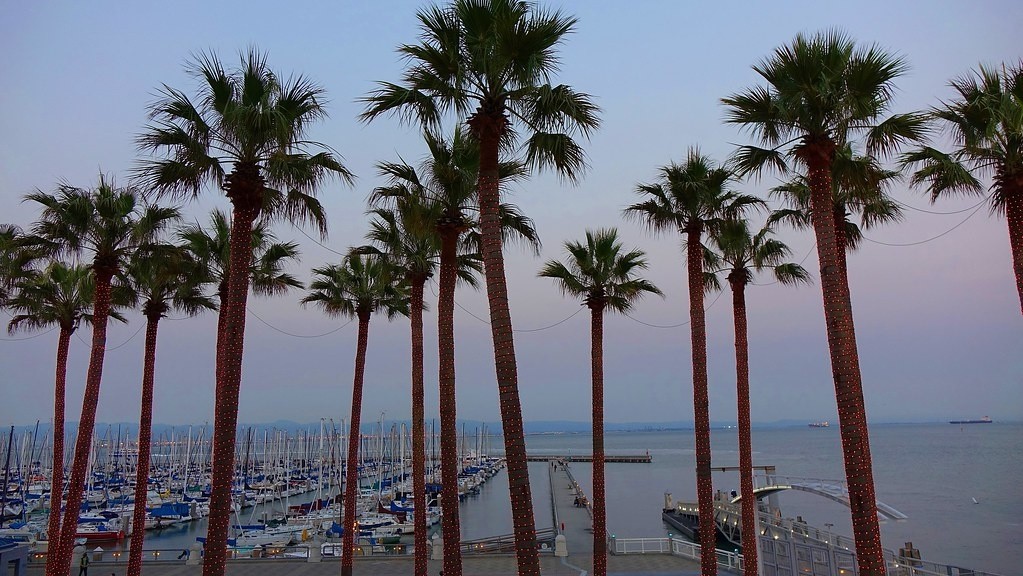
[949, 416, 992, 424]
[808, 422, 830, 431]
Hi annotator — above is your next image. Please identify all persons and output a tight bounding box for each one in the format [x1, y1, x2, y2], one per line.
[78, 553, 90, 576]
[553, 465, 557, 472]
[574, 496, 589, 508]
[551, 461, 554, 467]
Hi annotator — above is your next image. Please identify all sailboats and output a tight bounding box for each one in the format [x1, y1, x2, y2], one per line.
[0, 417, 508, 559]
